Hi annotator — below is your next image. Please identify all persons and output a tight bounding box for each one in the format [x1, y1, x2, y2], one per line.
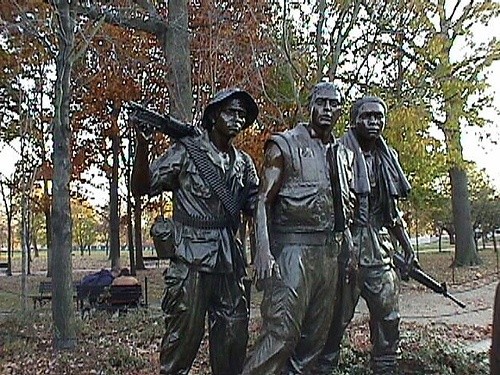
[90, 266, 121, 307]
[83, 267, 108, 285]
[131, 89, 260, 375]
[110, 268, 139, 307]
[308, 96, 421, 375]
[241, 82, 356, 375]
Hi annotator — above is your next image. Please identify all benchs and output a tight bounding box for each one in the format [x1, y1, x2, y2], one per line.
[142, 256, 160, 268]
[77, 284, 148, 319]
[32, 280, 84, 311]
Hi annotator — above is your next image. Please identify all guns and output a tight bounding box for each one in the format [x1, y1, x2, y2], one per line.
[125, 100, 201, 138]
[382, 244, 467, 309]
